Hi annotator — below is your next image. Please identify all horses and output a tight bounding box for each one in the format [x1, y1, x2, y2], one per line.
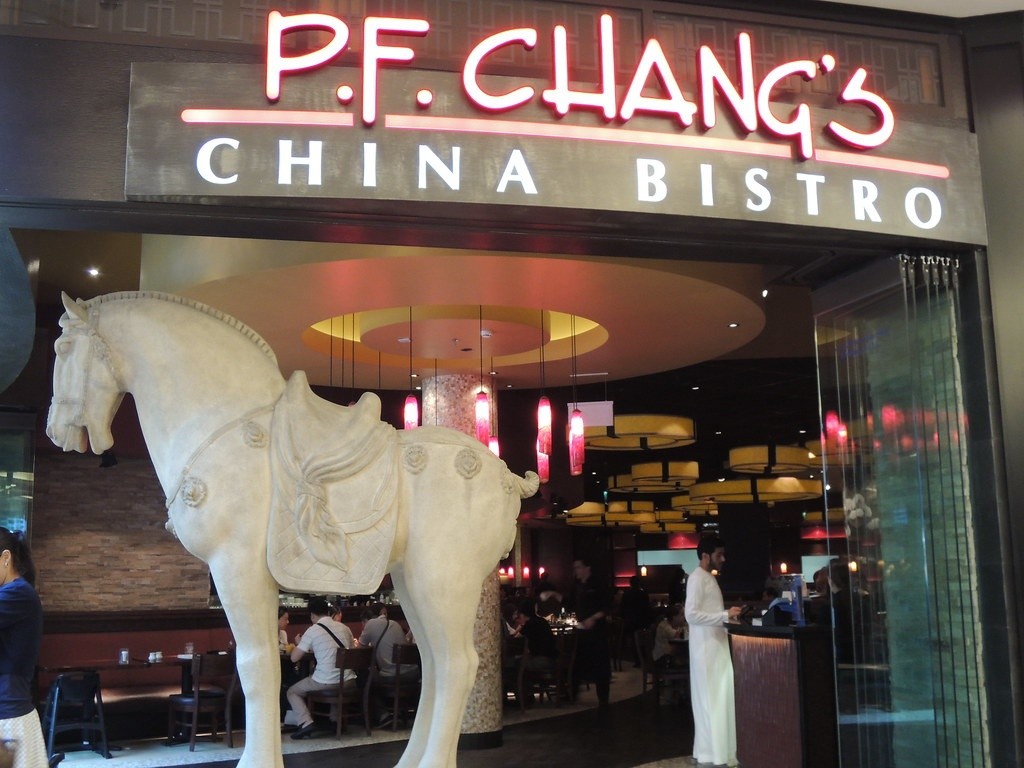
[45, 290, 540, 768]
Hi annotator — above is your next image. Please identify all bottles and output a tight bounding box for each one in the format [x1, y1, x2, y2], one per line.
[561, 607, 567, 624]
[571, 612, 577, 626]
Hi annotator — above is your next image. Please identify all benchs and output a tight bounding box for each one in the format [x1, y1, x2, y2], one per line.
[41, 623, 410, 713]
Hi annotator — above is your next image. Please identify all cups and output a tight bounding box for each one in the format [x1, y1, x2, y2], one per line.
[184, 642, 193, 656]
[155, 652, 163, 662]
[118, 648, 130, 665]
[148, 653, 156, 664]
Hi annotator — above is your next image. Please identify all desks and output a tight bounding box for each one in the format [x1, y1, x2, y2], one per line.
[132, 652, 236, 746]
[280, 653, 314, 707]
[37, 655, 149, 759]
[670, 639, 689, 647]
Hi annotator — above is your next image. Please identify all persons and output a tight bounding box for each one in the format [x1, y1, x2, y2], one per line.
[0, 527, 50, 768]
[278, 556, 867, 739]
[683, 535, 746, 767]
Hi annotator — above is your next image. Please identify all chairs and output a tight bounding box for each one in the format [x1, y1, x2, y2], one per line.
[372, 643, 422, 732]
[518, 624, 577, 713]
[607, 615, 655, 714]
[307, 647, 377, 740]
[42, 671, 112, 759]
[169, 654, 237, 752]
[635, 622, 690, 705]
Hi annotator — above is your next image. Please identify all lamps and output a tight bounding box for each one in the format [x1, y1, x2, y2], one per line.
[536, 309, 552, 483]
[475, 304, 490, 447]
[489, 355, 500, 457]
[569, 313, 585, 475]
[566, 415, 876, 533]
[404, 307, 418, 430]
